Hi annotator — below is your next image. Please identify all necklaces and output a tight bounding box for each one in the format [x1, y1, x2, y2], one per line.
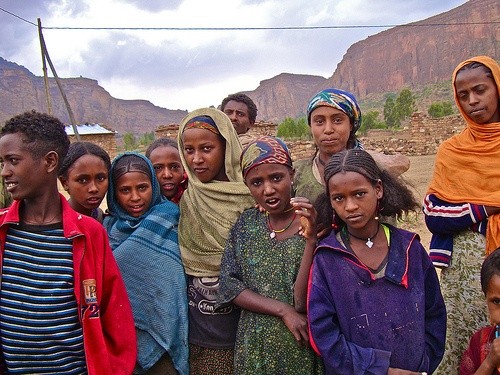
[318, 152, 326, 166]
[265, 206, 298, 240]
[342, 222, 381, 249]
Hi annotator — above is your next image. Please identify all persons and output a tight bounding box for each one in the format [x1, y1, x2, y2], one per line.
[423, 55, 500, 374]
[177, 107, 266, 375]
[220, 94, 259, 152]
[306, 149, 447, 374]
[57, 141, 112, 226]
[102, 150, 189, 375]
[145, 136, 189, 207]
[459, 246, 499, 374]
[0, 109, 137, 375]
[214, 136, 325, 375]
[291, 87, 367, 241]
